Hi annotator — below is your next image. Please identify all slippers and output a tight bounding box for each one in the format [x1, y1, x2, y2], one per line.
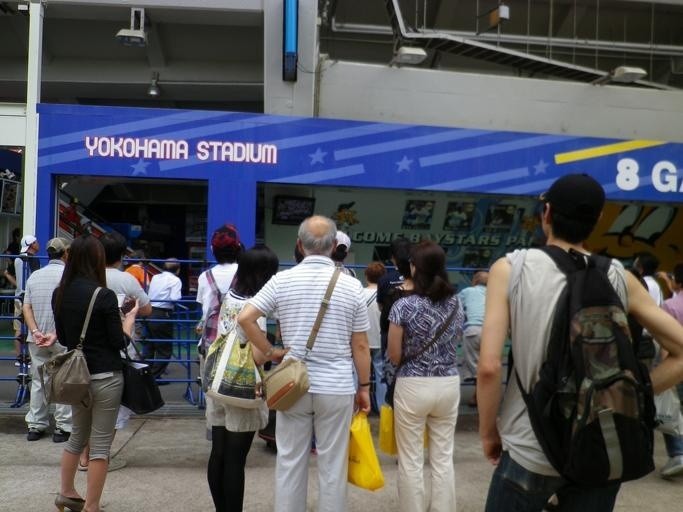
[78, 461, 88, 471]
[107, 459, 127, 473]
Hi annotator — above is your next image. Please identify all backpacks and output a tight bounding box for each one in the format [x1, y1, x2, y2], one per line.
[514, 243, 654, 484]
[203, 269, 237, 354]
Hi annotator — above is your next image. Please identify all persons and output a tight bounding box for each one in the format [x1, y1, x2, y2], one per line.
[332, 230, 356, 277]
[363, 262, 391, 415]
[624, 250, 683, 477]
[376, 239, 411, 359]
[1, 232, 182, 472]
[455, 272, 489, 406]
[51, 233, 139, 512]
[207, 245, 279, 512]
[476, 173, 683, 512]
[238, 215, 372, 512]
[196, 223, 239, 441]
[380, 245, 414, 337]
[387, 242, 465, 512]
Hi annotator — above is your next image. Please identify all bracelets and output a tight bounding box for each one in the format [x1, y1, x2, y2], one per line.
[358, 382, 371, 387]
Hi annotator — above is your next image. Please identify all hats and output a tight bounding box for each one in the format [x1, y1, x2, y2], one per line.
[21, 235, 36, 252]
[45, 238, 69, 254]
[69, 197, 78, 203]
[536, 173, 605, 217]
[213, 224, 239, 248]
[128, 250, 143, 259]
[334, 231, 351, 252]
[80, 218, 90, 227]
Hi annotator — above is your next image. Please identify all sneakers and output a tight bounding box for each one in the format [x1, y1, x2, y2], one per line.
[659, 455, 683, 477]
[27, 428, 46, 441]
[52, 427, 72, 443]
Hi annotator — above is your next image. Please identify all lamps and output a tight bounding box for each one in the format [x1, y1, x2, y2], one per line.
[387, 46, 428, 67]
[116, 6, 150, 49]
[146, 71, 162, 97]
[592, 66, 647, 86]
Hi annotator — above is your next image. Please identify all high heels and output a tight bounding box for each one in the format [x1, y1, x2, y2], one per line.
[55, 495, 85, 512]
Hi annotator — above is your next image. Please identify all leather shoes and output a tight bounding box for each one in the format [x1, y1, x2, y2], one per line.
[155, 376, 169, 384]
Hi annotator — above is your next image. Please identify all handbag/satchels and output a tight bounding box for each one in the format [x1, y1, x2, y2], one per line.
[202, 330, 264, 411]
[38, 349, 94, 410]
[385, 378, 396, 407]
[264, 357, 311, 410]
[121, 359, 165, 415]
